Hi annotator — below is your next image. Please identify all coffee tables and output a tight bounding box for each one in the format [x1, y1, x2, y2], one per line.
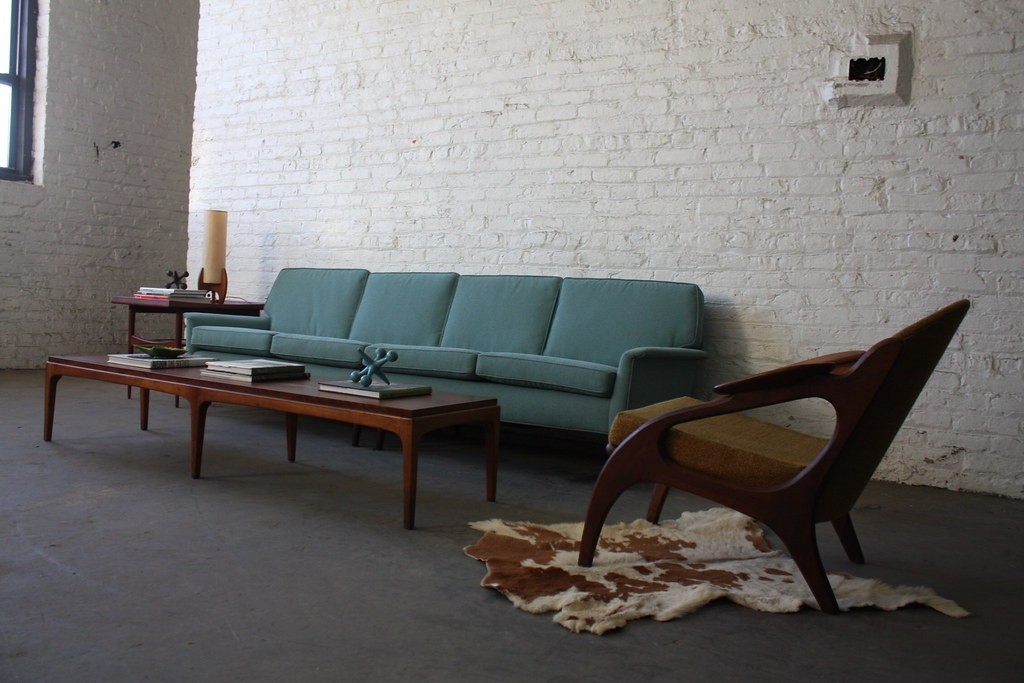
[42, 353, 501, 530]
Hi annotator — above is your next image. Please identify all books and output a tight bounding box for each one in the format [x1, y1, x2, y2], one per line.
[318, 380, 432, 399]
[200, 359, 310, 382]
[107, 354, 220, 368]
[134, 286, 211, 303]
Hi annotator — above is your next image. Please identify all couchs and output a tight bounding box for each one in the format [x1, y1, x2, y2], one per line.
[184, 267, 708, 482]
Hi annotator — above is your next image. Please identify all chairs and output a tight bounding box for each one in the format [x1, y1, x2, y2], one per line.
[578, 299, 970, 614]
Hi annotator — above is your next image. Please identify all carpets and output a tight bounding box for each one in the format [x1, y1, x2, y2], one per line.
[461, 506, 969, 636]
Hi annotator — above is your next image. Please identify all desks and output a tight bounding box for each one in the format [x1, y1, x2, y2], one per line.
[111, 296, 266, 409]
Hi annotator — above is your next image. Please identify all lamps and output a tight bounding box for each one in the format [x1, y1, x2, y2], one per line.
[198, 209, 229, 302]
